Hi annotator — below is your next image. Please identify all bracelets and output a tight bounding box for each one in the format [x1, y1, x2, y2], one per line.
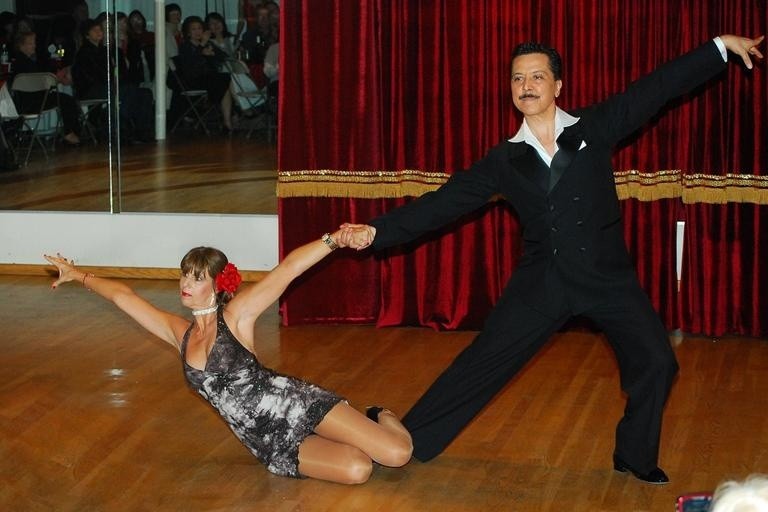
[82, 272, 95, 289]
[319, 231, 339, 252]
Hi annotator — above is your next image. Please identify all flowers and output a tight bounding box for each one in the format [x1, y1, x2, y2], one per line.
[214, 262, 242, 300]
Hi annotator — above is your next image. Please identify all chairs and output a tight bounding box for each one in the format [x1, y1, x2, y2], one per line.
[0, 19, 275, 169]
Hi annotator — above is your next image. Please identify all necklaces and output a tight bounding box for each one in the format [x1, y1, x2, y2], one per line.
[189, 305, 219, 317]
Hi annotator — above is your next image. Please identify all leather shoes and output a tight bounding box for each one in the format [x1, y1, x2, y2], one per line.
[364, 403, 401, 423]
[60, 139, 88, 148]
[611, 451, 673, 487]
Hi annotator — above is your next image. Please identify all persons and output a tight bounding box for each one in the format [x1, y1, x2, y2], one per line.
[329, 33, 768, 483]
[703, 474, 768, 511]
[42, 223, 415, 486]
[165, 1, 280, 136]
[1, 9, 155, 147]
